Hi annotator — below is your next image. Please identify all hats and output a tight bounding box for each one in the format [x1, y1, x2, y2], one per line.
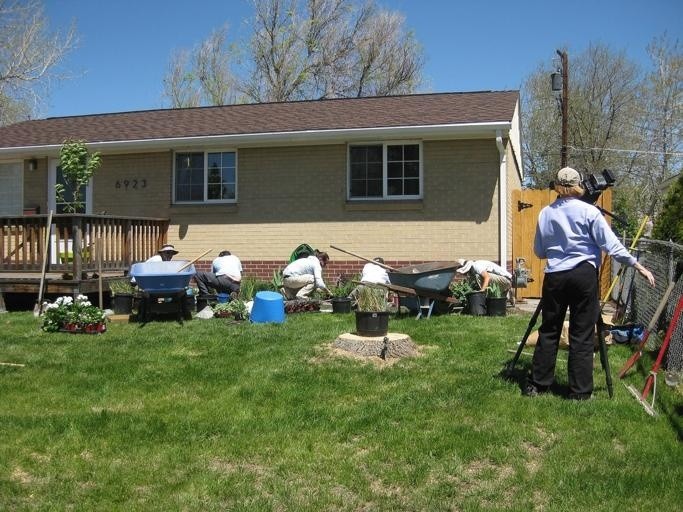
[456, 258, 474, 275]
[557, 166, 582, 188]
[157, 244, 179, 255]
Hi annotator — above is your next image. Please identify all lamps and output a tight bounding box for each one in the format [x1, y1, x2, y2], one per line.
[27, 158, 37, 171]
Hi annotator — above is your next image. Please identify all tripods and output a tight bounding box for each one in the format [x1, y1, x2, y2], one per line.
[505, 204, 613, 398]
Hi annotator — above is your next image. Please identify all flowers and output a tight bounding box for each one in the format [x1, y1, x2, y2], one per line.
[41, 292, 108, 334]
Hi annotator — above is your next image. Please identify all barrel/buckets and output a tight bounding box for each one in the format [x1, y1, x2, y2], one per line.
[464, 289, 488, 316]
[59, 252, 89, 279]
[249, 291, 285, 323]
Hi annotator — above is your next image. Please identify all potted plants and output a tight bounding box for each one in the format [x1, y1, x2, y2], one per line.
[351, 277, 391, 339]
[50, 133, 103, 265]
[485, 282, 506, 316]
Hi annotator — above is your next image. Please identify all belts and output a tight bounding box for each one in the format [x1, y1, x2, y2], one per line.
[283, 275, 289, 278]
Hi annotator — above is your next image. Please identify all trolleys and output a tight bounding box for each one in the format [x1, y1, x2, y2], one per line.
[351, 259, 461, 322]
[128, 259, 196, 322]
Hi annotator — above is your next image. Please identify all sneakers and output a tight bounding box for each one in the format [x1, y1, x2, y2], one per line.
[279, 287, 290, 301]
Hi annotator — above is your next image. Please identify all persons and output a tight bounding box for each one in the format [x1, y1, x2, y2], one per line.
[346, 257, 391, 308]
[523, 166, 656, 399]
[278, 249, 333, 300]
[130, 242, 178, 287]
[455, 258, 514, 306]
[192, 250, 242, 300]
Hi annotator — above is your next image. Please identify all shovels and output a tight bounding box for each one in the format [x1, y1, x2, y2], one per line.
[34, 210, 53, 317]
[618, 253, 682, 379]
[0, 295, 7, 312]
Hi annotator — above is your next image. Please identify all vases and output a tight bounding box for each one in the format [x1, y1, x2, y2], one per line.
[331, 297, 351, 313]
